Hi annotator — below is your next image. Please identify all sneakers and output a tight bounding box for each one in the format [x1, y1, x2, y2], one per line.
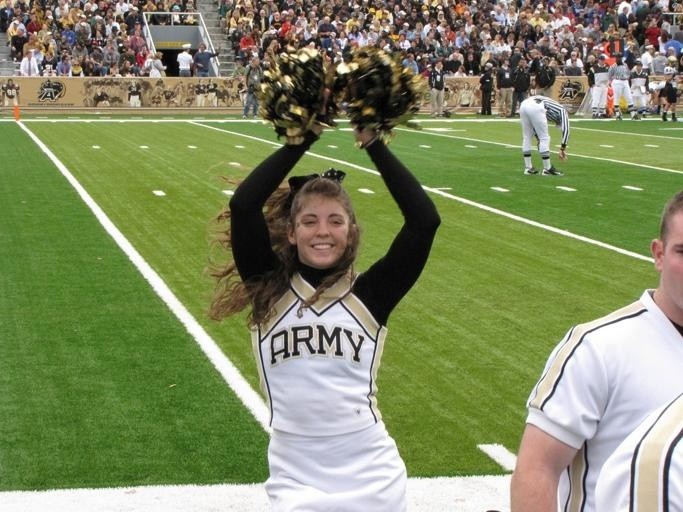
[592, 112, 679, 122]
[242, 112, 260, 120]
[542, 166, 562, 175]
[524, 168, 539, 174]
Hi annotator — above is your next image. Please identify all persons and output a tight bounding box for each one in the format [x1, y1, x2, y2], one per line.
[203, 124, 441, 511]
[522, 96, 569, 178]
[0, 1, 220, 108]
[216, 0, 683, 120]
[510, 193, 682, 511]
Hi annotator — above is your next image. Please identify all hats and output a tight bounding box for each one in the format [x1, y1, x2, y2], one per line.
[182, 44, 192, 50]
